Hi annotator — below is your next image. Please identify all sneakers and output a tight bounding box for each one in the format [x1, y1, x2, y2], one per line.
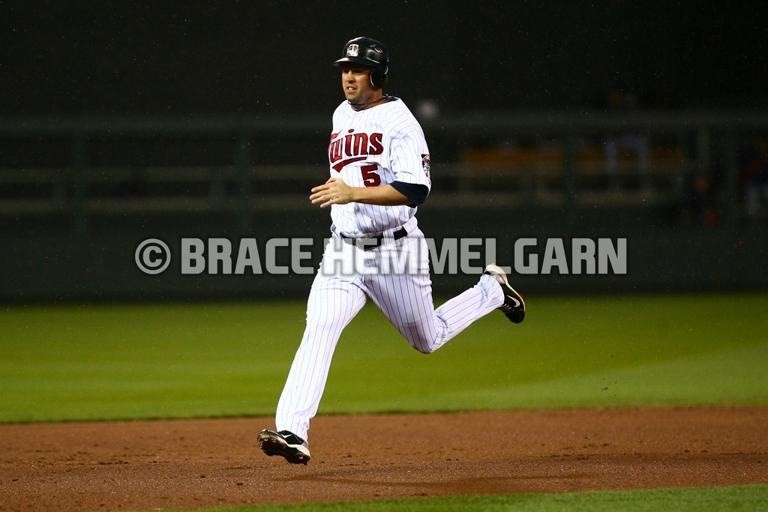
[250, 427, 311, 465]
[482, 263, 528, 323]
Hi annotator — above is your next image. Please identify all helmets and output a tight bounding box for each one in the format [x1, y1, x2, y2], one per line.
[332, 33, 389, 90]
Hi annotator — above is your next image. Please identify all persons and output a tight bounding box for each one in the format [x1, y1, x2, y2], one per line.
[257, 36, 525, 464]
